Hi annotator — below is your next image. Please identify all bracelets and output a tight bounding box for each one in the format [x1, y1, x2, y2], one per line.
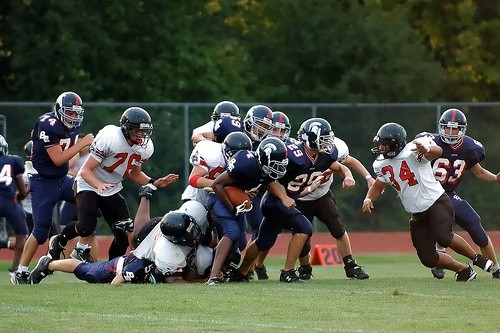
[308, 186, 312, 192]
[426, 146, 431, 153]
[364, 198, 371, 202]
[148, 178, 156, 183]
[365, 174, 371, 180]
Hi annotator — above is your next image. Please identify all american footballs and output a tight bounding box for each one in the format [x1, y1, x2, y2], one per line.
[224, 186, 251, 210]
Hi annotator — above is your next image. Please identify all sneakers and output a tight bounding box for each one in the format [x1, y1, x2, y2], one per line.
[431, 249, 445, 280]
[492, 268, 500, 280]
[343, 261, 370, 280]
[454, 264, 477, 283]
[112, 218, 134, 233]
[8, 235, 312, 285]
[472, 253, 493, 274]
[138, 183, 157, 199]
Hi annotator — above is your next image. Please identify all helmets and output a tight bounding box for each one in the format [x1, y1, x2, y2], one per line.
[243, 104, 273, 142]
[211, 101, 239, 123]
[0, 134, 8, 154]
[297, 117, 334, 154]
[255, 136, 287, 179]
[55, 92, 84, 129]
[439, 109, 468, 145]
[265, 111, 291, 142]
[370, 122, 407, 161]
[24, 142, 34, 159]
[120, 106, 153, 149]
[162, 211, 202, 249]
[222, 131, 253, 163]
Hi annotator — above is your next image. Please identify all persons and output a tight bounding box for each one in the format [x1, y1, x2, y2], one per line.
[362, 109, 500, 282]
[0, 92, 385, 285]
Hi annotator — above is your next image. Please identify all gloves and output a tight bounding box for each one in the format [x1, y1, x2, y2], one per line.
[233, 199, 253, 217]
